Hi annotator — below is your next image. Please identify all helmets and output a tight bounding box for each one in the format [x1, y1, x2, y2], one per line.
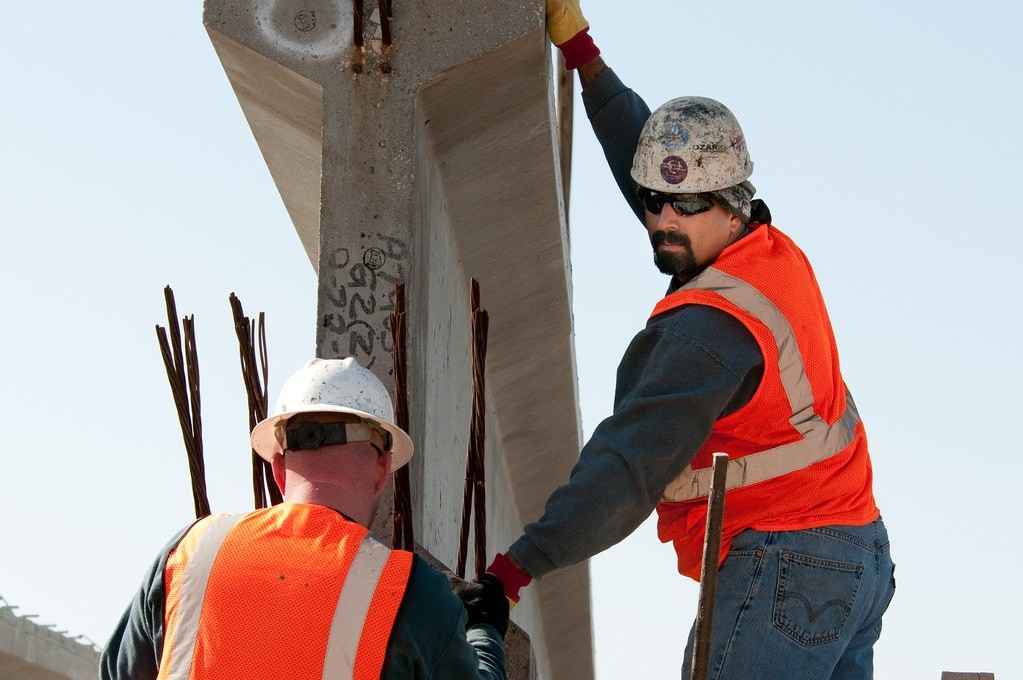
[630, 96, 753, 193]
[250, 357, 415, 474]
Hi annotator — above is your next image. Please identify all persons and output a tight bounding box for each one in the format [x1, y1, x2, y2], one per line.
[97, 357, 508, 680]
[463, 0, 896, 680]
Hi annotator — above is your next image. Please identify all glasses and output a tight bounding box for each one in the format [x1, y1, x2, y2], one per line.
[643, 190, 716, 216]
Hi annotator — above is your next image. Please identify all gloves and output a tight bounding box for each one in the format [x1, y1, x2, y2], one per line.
[443, 571, 509, 639]
[545, 0, 601, 72]
[483, 554, 531, 611]
